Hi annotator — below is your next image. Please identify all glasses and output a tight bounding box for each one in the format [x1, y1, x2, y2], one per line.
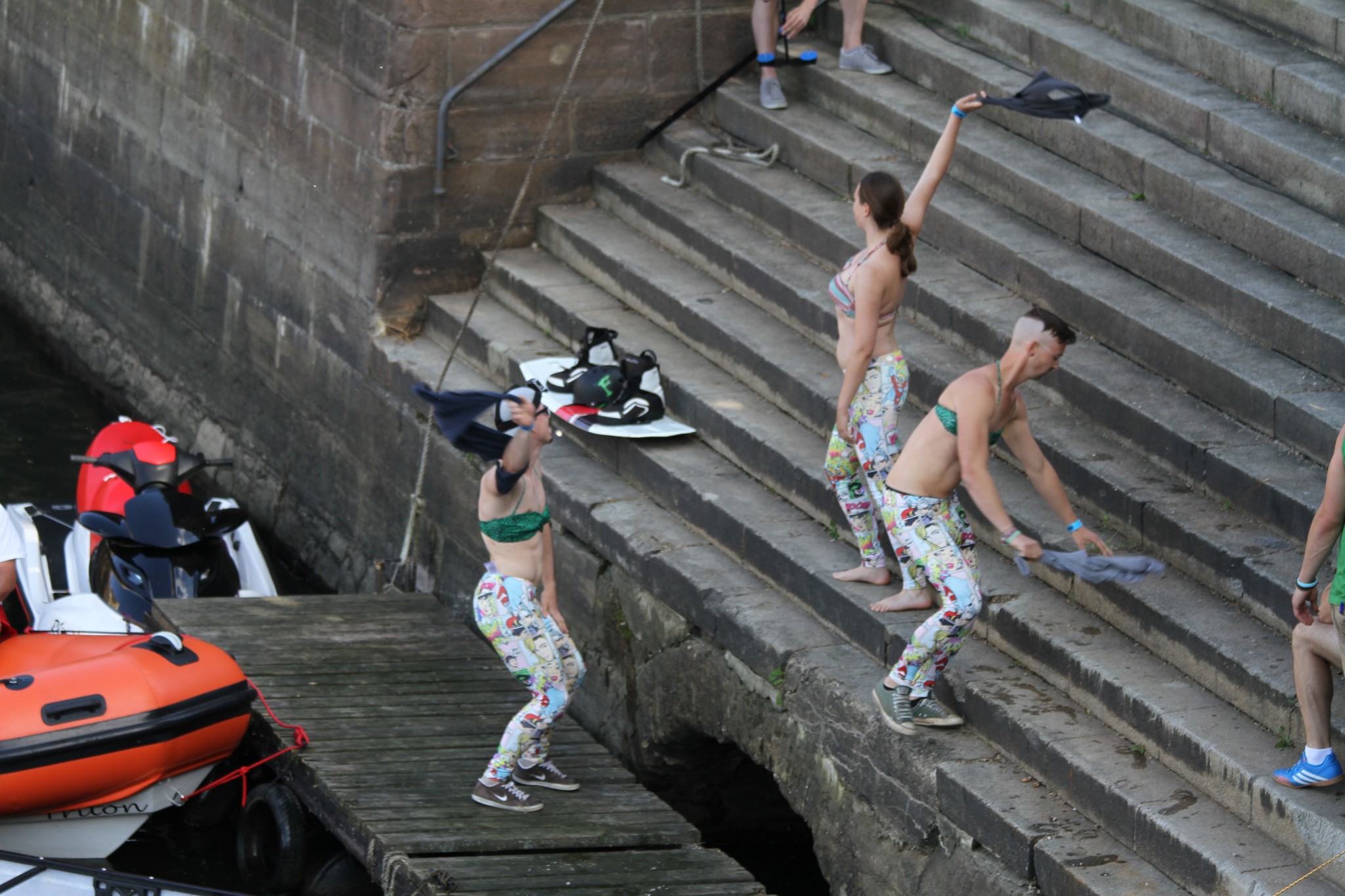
[534, 405, 551, 417]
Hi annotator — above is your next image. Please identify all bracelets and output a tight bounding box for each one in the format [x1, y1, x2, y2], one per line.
[1067, 520, 1084, 532]
[1295, 576, 1319, 591]
[521, 422, 535, 430]
[1004, 531, 1021, 544]
[952, 105, 970, 120]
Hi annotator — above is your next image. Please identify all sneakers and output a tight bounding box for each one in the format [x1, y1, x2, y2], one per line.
[839, 43, 892, 75]
[911, 690, 964, 726]
[471, 776, 544, 812]
[597, 349, 666, 425]
[547, 326, 620, 393]
[872, 679, 918, 736]
[760, 77, 788, 110]
[1272, 750, 1344, 788]
[512, 754, 581, 790]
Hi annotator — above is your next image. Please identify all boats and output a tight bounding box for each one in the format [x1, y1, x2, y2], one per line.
[1, 621, 260, 896]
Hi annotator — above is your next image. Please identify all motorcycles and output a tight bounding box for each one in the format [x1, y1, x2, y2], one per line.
[50, 415, 253, 633]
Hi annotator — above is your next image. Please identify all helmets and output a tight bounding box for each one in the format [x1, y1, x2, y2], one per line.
[573, 365, 630, 409]
[493, 387, 540, 437]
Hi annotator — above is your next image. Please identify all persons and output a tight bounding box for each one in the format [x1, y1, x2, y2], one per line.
[1291, 424, 1345, 679]
[751, 0, 893, 110]
[874, 306, 1113, 735]
[1272, 582, 1345, 789]
[824, 90, 987, 612]
[469, 383, 586, 813]
